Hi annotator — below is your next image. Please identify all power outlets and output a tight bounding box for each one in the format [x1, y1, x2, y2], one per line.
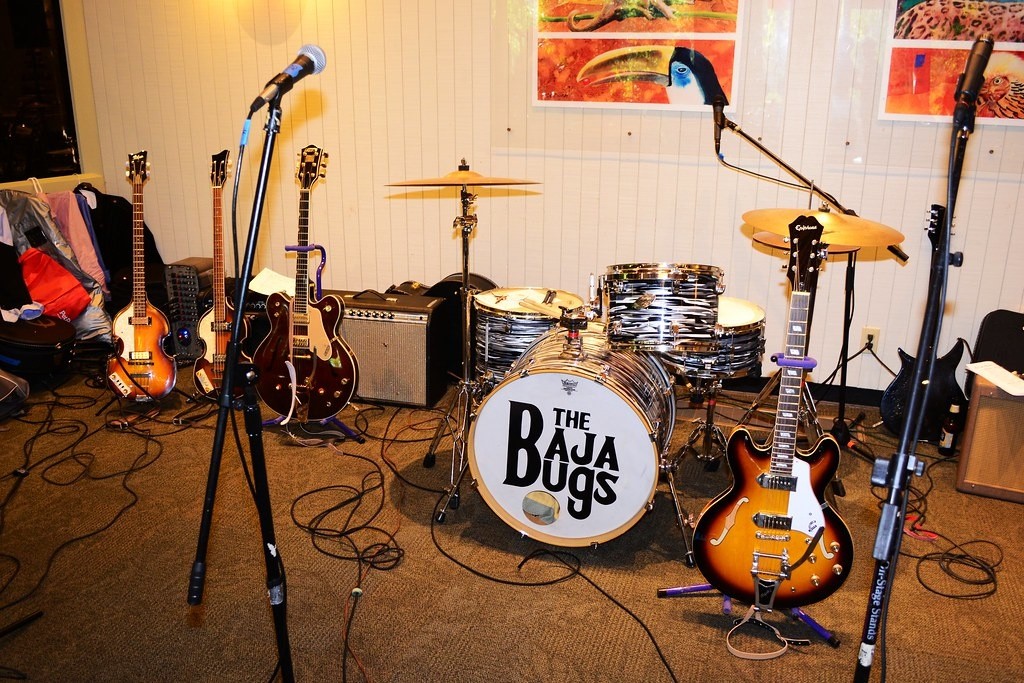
[860, 326, 880, 354]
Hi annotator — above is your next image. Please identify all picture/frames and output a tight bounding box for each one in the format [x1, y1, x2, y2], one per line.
[532, 0, 745, 114]
[876, 0, 1024, 126]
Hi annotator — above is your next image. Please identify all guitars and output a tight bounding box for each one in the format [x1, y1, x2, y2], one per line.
[252, 145, 360, 421]
[693, 215, 854, 608]
[193, 150, 254, 400]
[105, 151, 176, 402]
[880, 204, 966, 437]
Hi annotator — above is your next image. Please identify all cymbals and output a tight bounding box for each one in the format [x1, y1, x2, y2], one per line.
[753, 230, 861, 254]
[384, 172, 542, 186]
[741, 208, 905, 247]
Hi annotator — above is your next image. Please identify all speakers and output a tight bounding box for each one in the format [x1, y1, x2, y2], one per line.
[955, 374, 1024, 503]
[313, 290, 449, 408]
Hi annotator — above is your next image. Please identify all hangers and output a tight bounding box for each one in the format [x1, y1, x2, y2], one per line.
[26, 176, 43, 194]
[71, 172, 87, 192]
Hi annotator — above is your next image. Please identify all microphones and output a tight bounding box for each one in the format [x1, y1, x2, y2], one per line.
[250, 43, 326, 111]
[713, 94, 724, 150]
[953, 33, 995, 128]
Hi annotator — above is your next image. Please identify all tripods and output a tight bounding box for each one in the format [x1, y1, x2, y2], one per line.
[422, 157, 484, 507]
[672, 181, 846, 496]
[713, 114, 908, 459]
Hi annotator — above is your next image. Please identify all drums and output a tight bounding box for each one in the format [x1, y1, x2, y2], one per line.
[467, 320, 676, 547]
[473, 288, 584, 408]
[599, 263, 726, 352]
[659, 295, 765, 379]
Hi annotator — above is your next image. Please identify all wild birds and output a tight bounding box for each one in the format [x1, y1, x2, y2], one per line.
[974, 51, 1024, 119]
[577, 45, 730, 107]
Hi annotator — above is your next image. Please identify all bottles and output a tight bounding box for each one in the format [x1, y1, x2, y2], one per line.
[937, 401, 960, 456]
